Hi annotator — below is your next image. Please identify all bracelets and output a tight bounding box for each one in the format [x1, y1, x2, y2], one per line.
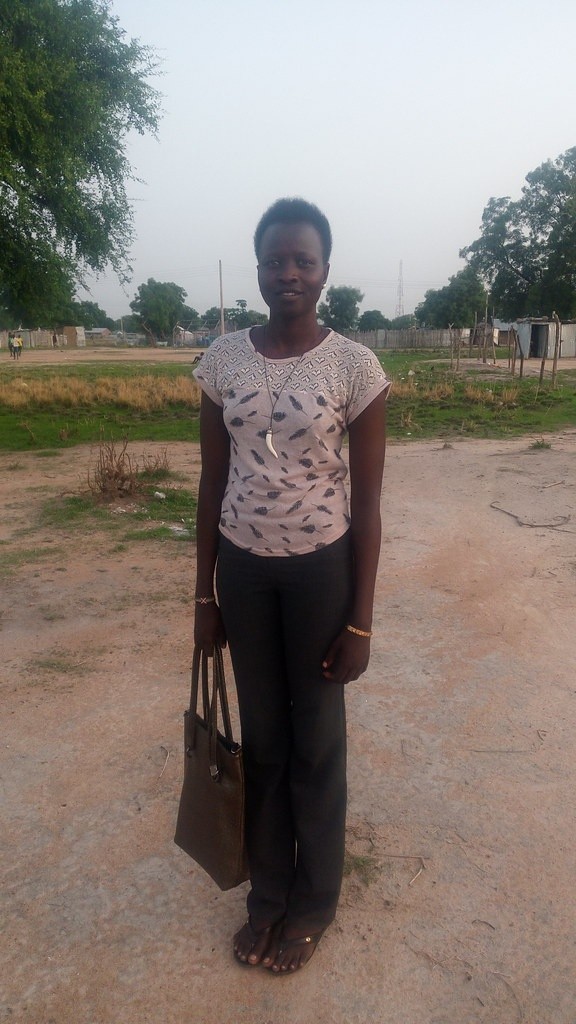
[194, 596, 215, 604]
[344, 622, 374, 638]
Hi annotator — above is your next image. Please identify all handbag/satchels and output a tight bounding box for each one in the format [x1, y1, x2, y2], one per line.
[174, 637, 254, 893]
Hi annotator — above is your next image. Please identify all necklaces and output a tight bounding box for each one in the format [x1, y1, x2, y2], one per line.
[263, 324, 324, 460]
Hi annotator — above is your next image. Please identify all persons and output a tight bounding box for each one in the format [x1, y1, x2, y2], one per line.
[8, 332, 24, 360]
[188, 198, 392, 976]
